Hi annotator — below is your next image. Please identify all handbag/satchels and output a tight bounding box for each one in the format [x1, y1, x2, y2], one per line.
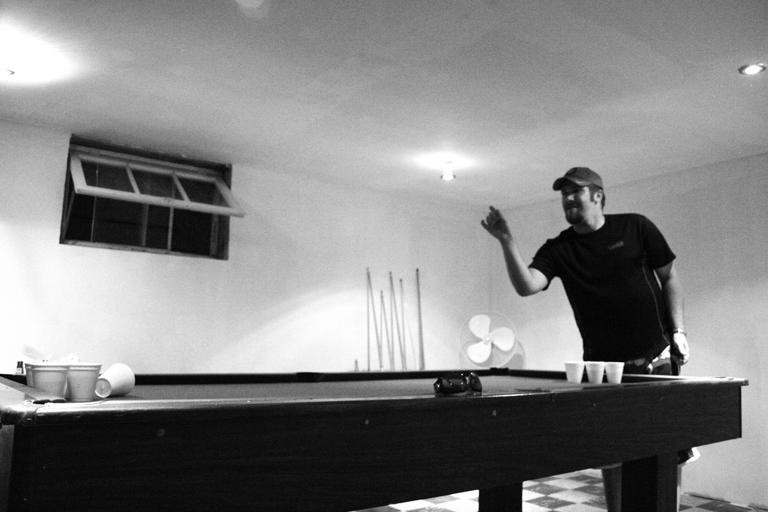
[672, 326, 689, 338]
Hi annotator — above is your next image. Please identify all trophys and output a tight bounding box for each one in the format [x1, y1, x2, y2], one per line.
[415, 267, 425, 371]
[399, 278, 407, 371]
[367, 271, 382, 373]
[389, 272, 404, 372]
[382, 292, 393, 372]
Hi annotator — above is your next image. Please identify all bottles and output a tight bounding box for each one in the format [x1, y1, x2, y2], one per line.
[552, 165, 604, 193]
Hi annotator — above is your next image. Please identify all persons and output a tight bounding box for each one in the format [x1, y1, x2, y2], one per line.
[479, 165, 702, 512]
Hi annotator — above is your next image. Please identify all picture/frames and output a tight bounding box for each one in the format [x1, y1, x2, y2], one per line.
[0, 366, 750, 512]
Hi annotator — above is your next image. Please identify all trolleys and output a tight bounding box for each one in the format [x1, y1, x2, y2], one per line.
[460, 310, 519, 369]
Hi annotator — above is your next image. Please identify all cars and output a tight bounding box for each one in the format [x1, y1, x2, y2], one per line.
[605, 362, 626, 385]
[67, 364, 101, 402]
[32, 364, 70, 397]
[25, 363, 33, 387]
[565, 362, 585, 384]
[586, 361, 605, 386]
[95, 363, 136, 399]
